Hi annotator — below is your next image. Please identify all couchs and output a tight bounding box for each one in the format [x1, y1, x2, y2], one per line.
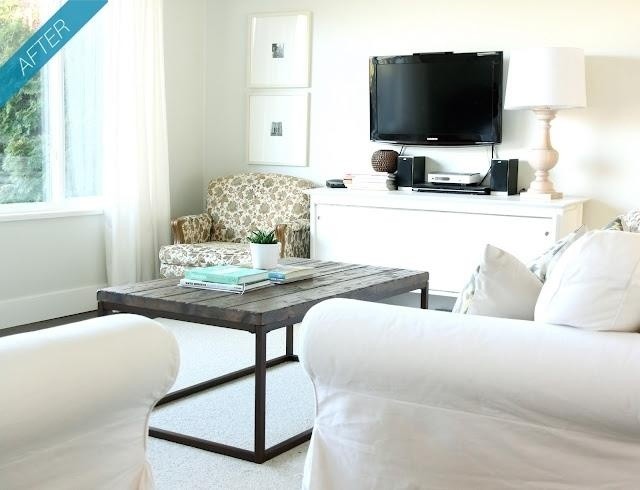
[159, 171, 315, 279]
[298, 208, 640, 490]
[0, 314, 179, 490]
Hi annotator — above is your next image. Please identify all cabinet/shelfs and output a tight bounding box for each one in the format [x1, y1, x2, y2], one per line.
[301, 182, 590, 300]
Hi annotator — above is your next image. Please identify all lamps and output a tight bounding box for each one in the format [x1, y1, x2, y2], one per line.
[505, 45, 588, 201]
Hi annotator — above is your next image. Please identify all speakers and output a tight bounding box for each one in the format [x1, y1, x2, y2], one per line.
[491, 159, 518, 195]
[397, 156, 426, 186]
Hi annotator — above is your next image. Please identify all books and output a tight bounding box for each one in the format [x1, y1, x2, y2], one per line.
[343, 172, 397, 191]
[178, 264, 317, 295]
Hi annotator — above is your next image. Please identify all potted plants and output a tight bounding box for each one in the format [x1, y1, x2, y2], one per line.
[247, 230, 283, 270]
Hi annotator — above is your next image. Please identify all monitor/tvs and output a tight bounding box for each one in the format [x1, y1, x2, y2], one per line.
[369, 51, 503, 144]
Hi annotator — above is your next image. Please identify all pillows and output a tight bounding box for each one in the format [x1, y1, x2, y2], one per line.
[450, 242, 541, 319]
[601, 216, 628, 232]
[529, 223, 588, 286]
[534, 227, 639, 333]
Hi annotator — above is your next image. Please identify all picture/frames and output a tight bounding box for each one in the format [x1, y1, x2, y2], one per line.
[245, 92, 312, 167]
[248, 10, 314, 90]
